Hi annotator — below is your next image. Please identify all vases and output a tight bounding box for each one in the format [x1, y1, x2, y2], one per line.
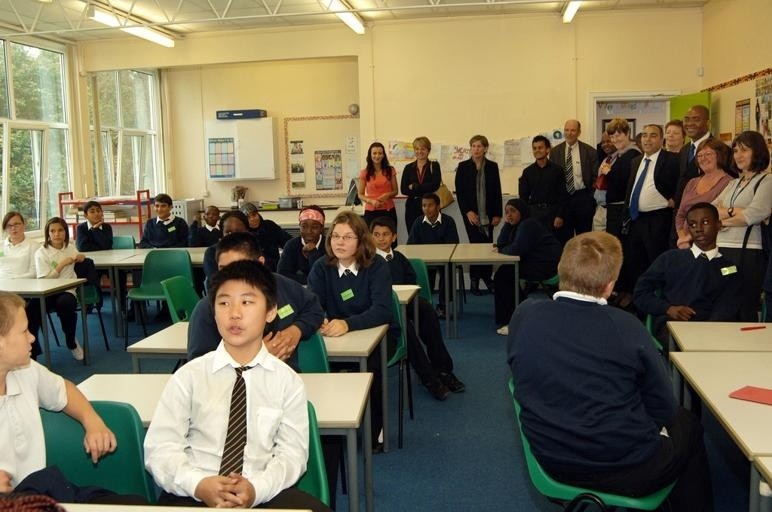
[237, 197, 244, 209]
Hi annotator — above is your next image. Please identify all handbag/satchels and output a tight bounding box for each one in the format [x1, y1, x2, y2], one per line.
[595, 160, 611, 190]
[434, 184, 454, 209]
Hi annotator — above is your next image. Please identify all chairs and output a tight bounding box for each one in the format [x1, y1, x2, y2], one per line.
[0, 227, 435, 512]
[508, 245, 771, 512]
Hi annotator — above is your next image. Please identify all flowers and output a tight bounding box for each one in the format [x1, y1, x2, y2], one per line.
[228, 182, 250, 201]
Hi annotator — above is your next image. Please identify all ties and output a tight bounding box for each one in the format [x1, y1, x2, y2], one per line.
[220, 367, 253, 477]
[345, 269, 350, 276]
[687, 144, 695, 165]
[566, 147, 575, 195]
[386, 255, 392, 262]
[630, 159, 651, 220]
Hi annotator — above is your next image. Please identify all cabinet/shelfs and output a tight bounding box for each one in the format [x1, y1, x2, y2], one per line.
[200, 206, 351, 227]
[164, 198, 205, 225]
[58, 188, 153, 290]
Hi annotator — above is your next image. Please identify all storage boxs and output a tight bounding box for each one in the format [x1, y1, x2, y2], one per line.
[276, 194, 299, 209]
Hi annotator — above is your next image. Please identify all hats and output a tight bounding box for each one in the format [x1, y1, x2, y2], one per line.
[240, 203, 256, 215]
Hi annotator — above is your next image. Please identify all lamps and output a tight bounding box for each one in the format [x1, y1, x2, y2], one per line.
[559, 0, 583, 25]
[80, 0, 186, 50]
[317, 0, 370, 40]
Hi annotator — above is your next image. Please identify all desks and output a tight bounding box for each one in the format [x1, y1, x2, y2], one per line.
[393, 244, 458, 337]
[451, 243, 520, 337]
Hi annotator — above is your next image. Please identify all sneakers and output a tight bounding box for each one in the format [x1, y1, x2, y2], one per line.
[441, 372, 465, 392]
[71, 342, 84, 360]
[426, 379, 449, 400]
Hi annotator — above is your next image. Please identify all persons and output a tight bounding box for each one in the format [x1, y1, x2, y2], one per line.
[369, 218, 466, 402]
[143, 259, 335, 510]
[1, 291, 152, 505]
[507, 229, 711, 510]
[307, 212, 402, 453]
[455, 135, 503, 296]
[493, 105, 771, 335]
[401, 137, 442, 235]
[3, 202, 114, 361]
[405, 193, 459, 319]
[358, 142, 398, 251]
[125, 193, 327, 322]
[187, 232, 324, 361]
[632, 202, 759, 356]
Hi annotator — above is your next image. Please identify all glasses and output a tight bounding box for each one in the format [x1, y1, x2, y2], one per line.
[331, 232, 358, 241]
